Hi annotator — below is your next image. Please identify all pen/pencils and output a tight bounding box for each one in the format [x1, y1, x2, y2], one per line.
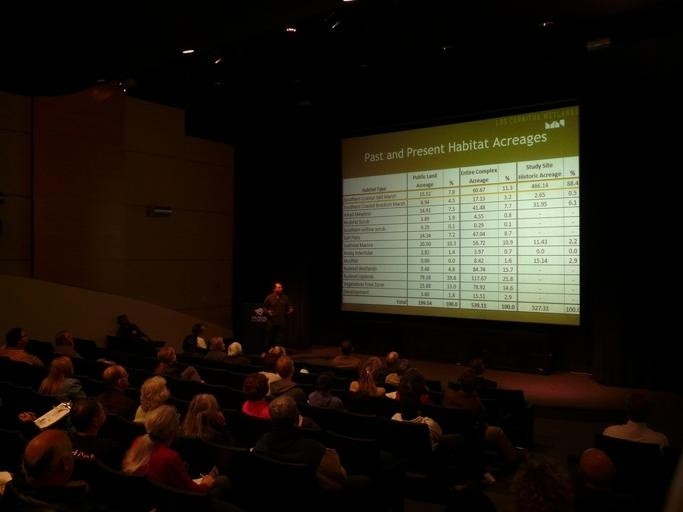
[200, 473, 204, 478]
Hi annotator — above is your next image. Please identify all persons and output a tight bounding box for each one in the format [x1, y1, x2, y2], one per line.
[0, 313, 683, 511]
[262, 283, 294, 355]
